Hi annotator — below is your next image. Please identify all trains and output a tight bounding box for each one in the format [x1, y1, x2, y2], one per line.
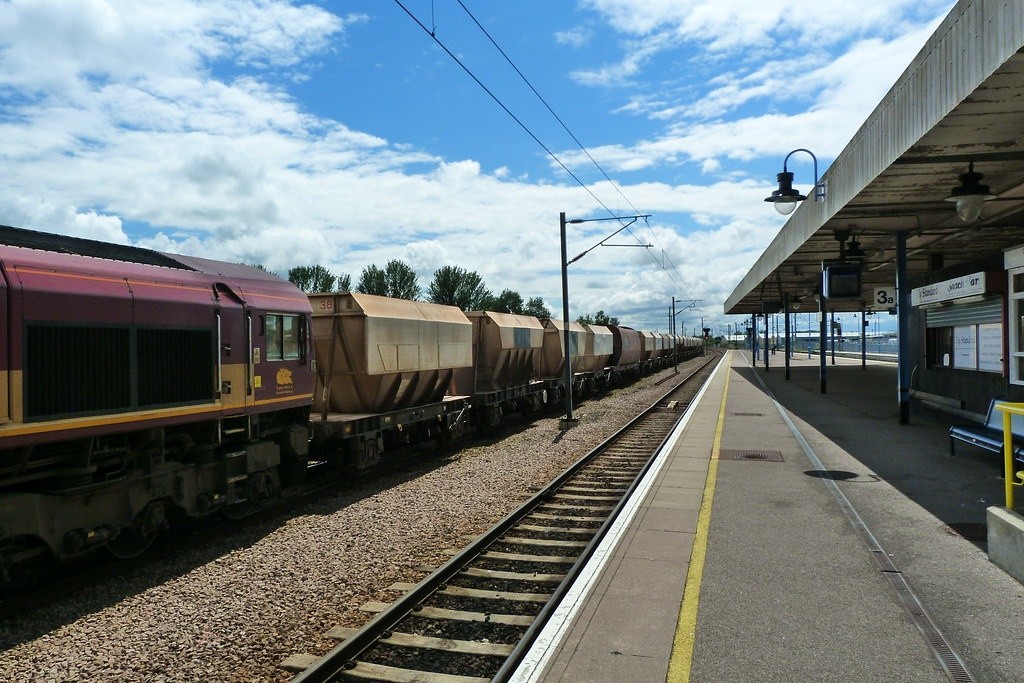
[1, 225, 702, 589]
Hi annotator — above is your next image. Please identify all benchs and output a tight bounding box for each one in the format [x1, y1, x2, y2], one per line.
[950, 396, 1024, 480]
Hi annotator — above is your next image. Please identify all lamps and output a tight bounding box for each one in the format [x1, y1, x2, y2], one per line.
[763, 148, 827, 214]
[944, 161, 1000, 226]
[845, 234, 867, 266]
[791, 291, 803, 311]
[738, 301, 784, 329]
